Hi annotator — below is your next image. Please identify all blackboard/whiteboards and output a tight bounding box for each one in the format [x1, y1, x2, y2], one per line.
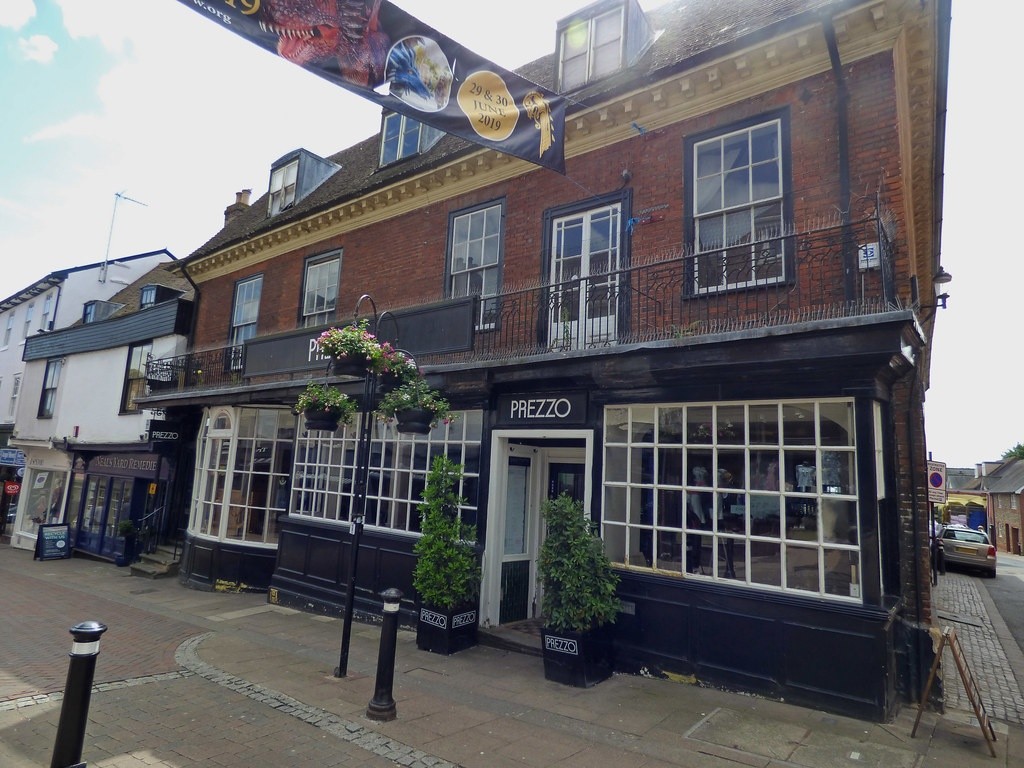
[35, 523, 70, 559]
[917, 625, 989, 734]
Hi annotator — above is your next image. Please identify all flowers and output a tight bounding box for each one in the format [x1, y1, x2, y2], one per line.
[371, 379, 461, 428]
[380, 352, 424, 383]
[294, 381, 358, 427]
[316, 318, 396, 376]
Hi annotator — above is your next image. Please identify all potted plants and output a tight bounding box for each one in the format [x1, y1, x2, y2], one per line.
[113, 520, 137, 566]
[413, 454, 483, 655]
[536, 489, 620, 690]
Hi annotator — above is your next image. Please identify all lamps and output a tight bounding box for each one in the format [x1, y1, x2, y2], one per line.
[919, 265, 953, 327]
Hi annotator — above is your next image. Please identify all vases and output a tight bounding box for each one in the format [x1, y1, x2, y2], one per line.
[395, 409, 434, 435]
[329, 353, 369, 378]
[304, 405, 341, 432]
[381, 371, 406, 386]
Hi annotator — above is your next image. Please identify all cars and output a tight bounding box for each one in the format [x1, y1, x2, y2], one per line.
[929, 518, 971, 537]
[928, 524, 997, 579]
